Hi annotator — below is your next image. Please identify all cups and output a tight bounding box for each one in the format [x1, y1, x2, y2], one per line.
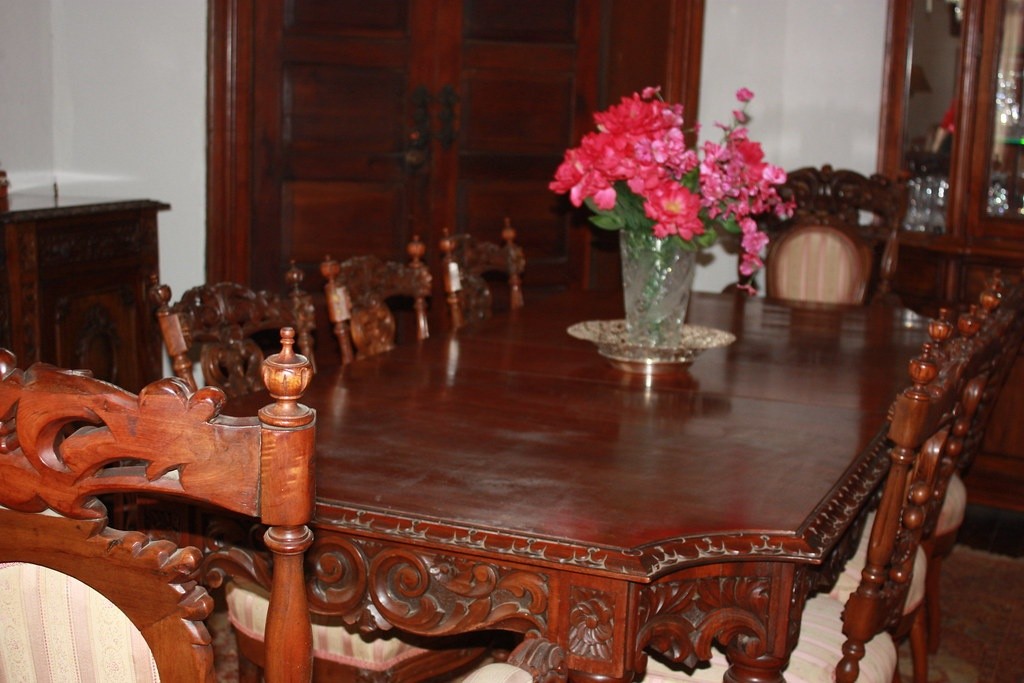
[898, 69, 1021, 236]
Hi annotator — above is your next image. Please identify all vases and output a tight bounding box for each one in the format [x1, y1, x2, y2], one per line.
[607, 207, 698, 344]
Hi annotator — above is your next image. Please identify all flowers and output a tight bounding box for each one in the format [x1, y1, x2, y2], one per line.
[546, 78, 798, 292]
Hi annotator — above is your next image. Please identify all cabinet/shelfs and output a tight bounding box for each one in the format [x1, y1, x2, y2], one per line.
[861, 0, 1024, 536]
[2, 195, 176, 381]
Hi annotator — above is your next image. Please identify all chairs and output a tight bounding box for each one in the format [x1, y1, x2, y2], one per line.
[440, 217, 528, 330]
[143, 255, 318, 412]
[751, 165, 912, 304]
[312, 231, 434, 359]
[2, 325, 319, 683]
[553, 274, 1024, 683]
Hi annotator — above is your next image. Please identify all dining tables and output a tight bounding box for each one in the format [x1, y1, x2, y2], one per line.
[155, 271, 989, 683]
